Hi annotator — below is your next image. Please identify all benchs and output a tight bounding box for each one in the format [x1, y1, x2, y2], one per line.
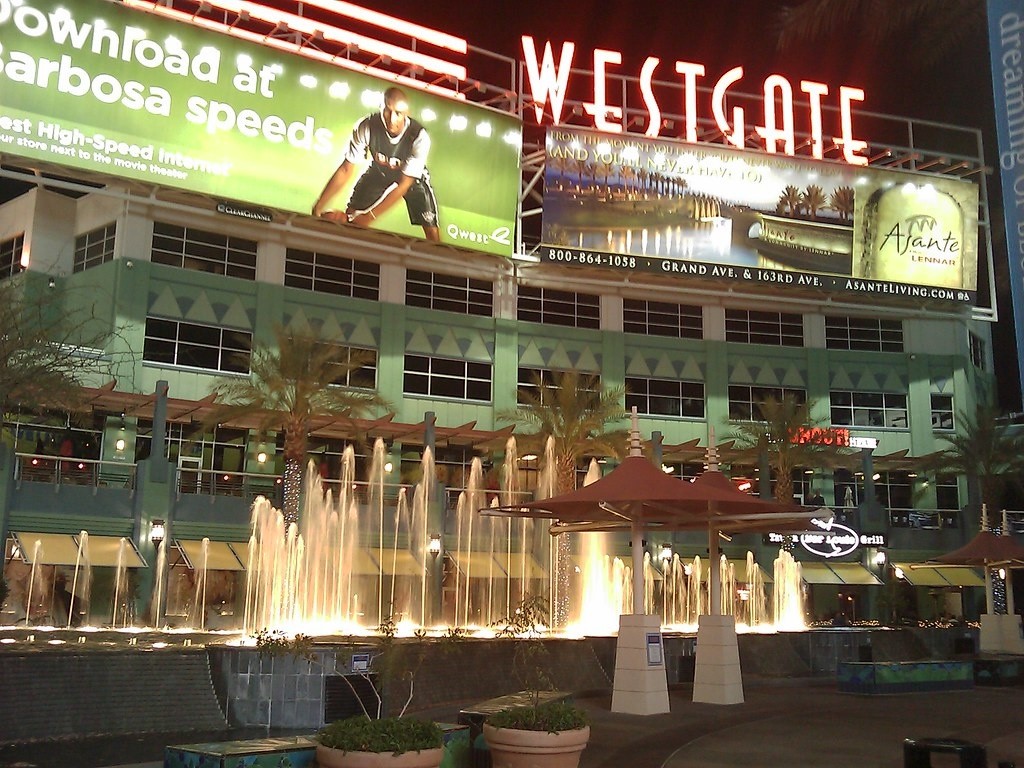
[974, 648, 1024, 686]
[840, 656, 1016, 691]
[163, 722, 471, 768]
[457, 689, 573, 768]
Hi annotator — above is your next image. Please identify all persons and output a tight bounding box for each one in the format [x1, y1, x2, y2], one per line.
[941, 613, 967, 622]
[312, 86, 442, 240]
[830, 609, 851, 627]
[805, 489, 826, 505]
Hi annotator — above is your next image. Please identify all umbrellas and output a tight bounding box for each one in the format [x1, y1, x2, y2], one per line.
[480, 455, 829, 712]
[924, 531, 1024, 655]
[843, 485, 854, 514]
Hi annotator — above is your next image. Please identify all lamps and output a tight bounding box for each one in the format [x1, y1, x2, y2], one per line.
[429, 534, 442, 559]
[876, 549, 885, 570]
[661, 543, 672, 565]
[152, 519, 166, 549]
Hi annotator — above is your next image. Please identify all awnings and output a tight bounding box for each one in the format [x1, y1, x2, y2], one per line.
[797, 562, 885, 585]
[8, 531, 150, 592]
[675, 553, 776, 586]
[891, 561, 988, 589]
[170, 541, 432, 609]
[445, 546, 549, 595]
[563, 549, 667, 586]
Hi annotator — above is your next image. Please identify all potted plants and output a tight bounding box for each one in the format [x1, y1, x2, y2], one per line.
[482, 591, 590, 768]
[248, 613, 466, 768]
